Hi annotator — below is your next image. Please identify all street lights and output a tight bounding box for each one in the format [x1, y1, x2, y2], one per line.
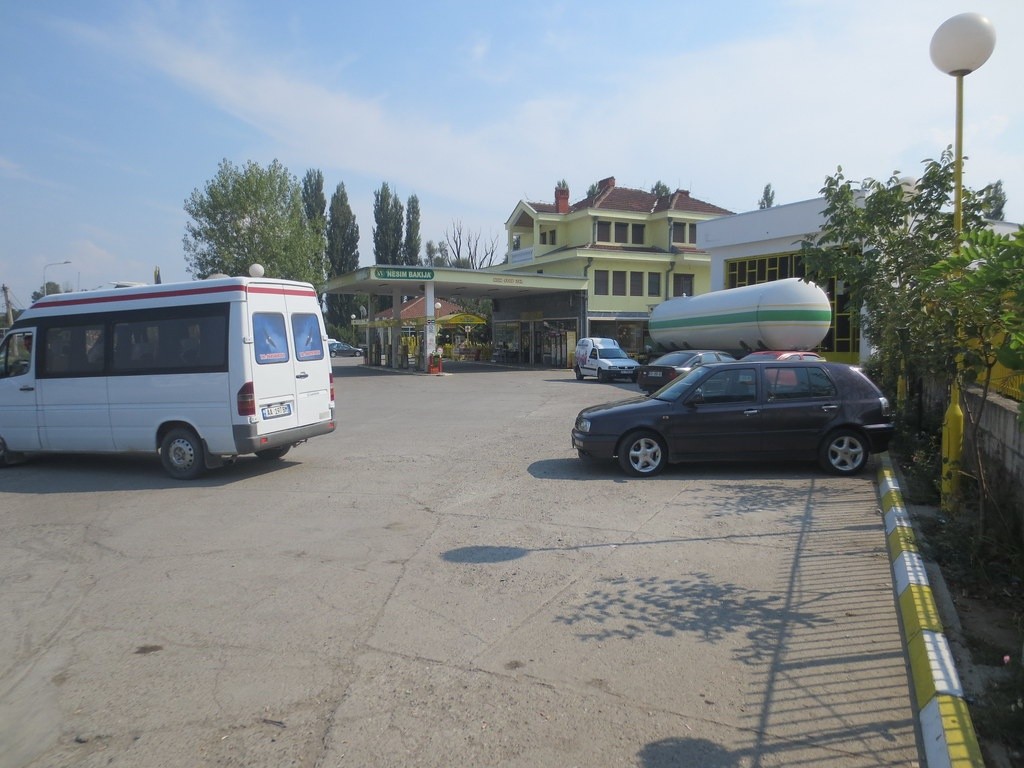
[928, 11, 998, 512]
[42, 260, 73, 296]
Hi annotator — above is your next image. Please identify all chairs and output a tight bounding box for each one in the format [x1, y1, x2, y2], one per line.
[46, 331, 200, 371]
[729, 381, 749, 400]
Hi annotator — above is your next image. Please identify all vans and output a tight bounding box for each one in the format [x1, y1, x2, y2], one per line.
[1, 276, 338, 480]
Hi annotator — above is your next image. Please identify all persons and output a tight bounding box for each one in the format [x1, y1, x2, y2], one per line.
[589, 350, 597, 359]
[10, 335, 32, 377]
[662, 368, 685, 383]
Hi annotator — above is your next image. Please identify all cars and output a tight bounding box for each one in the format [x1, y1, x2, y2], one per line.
[328, 342, 364, 357]
[725, 350, 827, 387]
[570, 362, 896, 477]
[573, 337, 640, 383]
[638, 349, 738, 394]
